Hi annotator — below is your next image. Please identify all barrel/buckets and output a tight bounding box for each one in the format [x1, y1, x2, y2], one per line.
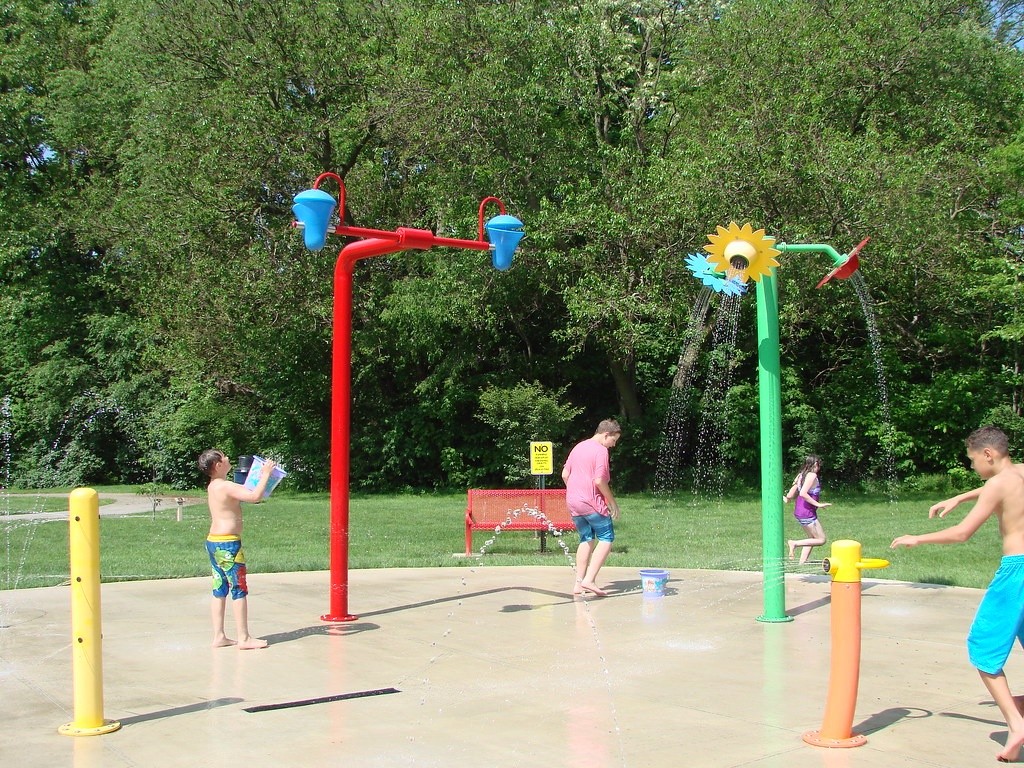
[639, 569, 670, 598]
[242, 455, 287, 501]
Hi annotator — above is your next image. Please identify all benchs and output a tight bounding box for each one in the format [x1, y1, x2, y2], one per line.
[465, 488, 608, 556]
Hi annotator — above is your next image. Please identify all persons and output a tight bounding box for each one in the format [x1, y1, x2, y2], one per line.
[889, 426, 1024, 761]
[783, 453, 832, 566]
[198, 448, 276, 651]
[562, 420, 621, 596]
